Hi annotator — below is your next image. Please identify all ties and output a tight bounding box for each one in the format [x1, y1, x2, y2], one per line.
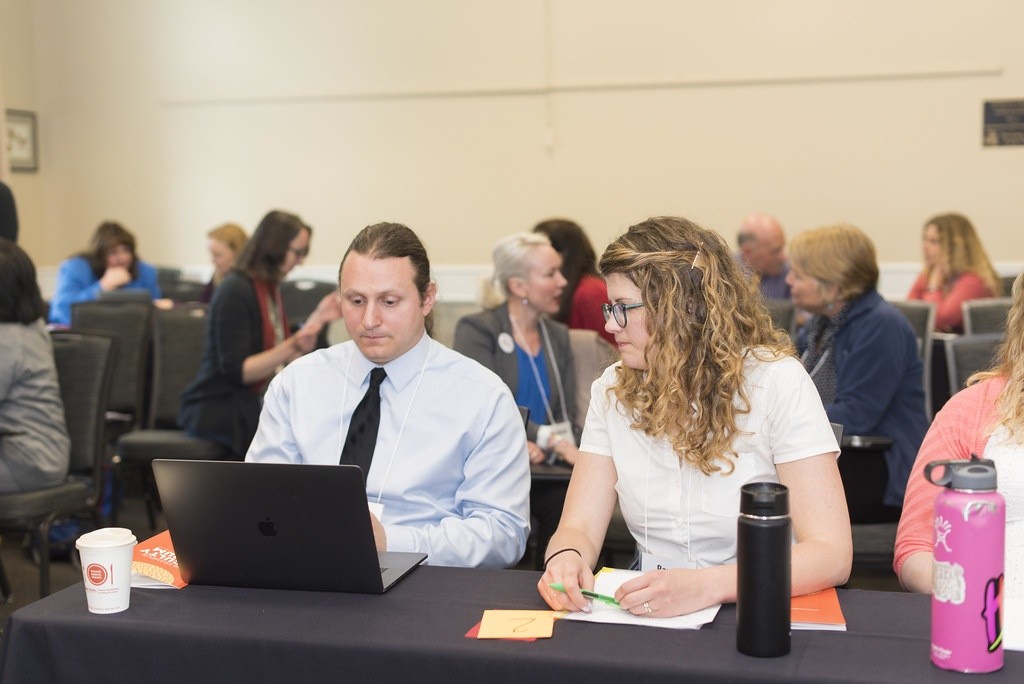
[340, 367, 387, 486]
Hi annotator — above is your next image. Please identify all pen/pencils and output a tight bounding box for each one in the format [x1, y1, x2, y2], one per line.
[547, 583, 622, 608]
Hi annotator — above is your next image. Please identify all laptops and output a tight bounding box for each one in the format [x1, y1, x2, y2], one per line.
[152, 459, 429, 593]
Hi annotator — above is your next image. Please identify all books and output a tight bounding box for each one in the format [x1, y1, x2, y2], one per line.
[130, 530, 187, 589]
[791, 587, 846, 631]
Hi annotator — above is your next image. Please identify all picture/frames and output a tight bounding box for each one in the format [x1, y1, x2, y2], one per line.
[7, 108, 38, 171]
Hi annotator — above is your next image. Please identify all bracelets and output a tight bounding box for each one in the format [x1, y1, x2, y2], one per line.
[543, 548, 582, 571]
[550, 435, 563, 450]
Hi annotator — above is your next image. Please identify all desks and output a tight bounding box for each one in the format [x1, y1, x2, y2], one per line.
[7, 565, 1024, 684]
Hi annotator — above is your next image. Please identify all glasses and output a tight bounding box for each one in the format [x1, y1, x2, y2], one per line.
[601, 301, 647, 328]
[289, 247, 306, 256]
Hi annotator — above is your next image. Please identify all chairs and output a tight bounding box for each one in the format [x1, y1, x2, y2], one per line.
[0, 270, 332, 598]
[748, 277, 1014, 552]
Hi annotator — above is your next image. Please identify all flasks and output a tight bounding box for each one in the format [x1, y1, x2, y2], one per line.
[924, 454, 1004, 674]
[738, 481, 791, 657]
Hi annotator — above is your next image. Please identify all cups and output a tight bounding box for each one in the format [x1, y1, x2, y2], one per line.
[75, 527, 138, 614]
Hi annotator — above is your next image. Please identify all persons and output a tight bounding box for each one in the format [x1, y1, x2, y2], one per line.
[452, 231, 582, 465]
[537, 216, 853, 618]
[171, 209, 343, 458]
[244, 220, 530, 568]
[737, 214, 808, 356]
[201, 225, 249, 303]
[0, 236, 69, 605]
[534, 218, 618, 348]
[0, 181, 19, 243]
[49, 221, 159, 325]
[783, 222, 930, 524]
[908, 212, 1005, 333]
[894, 272, 1024, 595]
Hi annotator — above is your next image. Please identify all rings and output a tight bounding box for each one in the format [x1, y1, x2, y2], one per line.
[644, 602, 651, 613]
[530, 451, 538, 458]
